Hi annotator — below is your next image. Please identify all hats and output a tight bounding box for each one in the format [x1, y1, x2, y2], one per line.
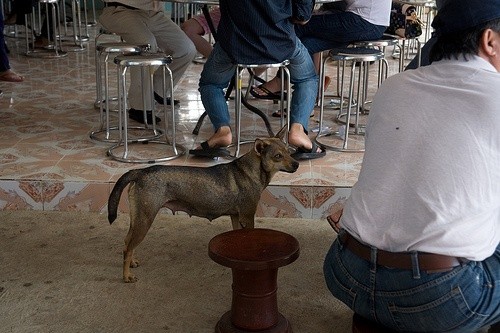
[431, 0, 500, 30]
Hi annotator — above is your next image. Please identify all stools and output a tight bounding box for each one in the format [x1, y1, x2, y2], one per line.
[222, 60, 292, 160]
[208, 228, 300, 333]
[313, 0, 438, 153]
[0, 0, 98, 59]
[90, 27, 187, 163]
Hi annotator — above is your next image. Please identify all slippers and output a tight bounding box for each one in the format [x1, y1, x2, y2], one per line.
[189, 141, 232, 157]
[250, 86, 288, 99]
[272, 107, 315, 119]
[293, 142, 326, 160]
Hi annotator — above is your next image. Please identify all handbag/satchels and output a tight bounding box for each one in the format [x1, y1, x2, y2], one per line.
[384, 2, 423, 40]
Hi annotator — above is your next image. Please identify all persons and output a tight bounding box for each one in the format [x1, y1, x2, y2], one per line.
[189, 0, 326, 159]
[249, 0, 393, 118]
[98, 0, 196, 125]
[323, 0, 500, 333]
[0, 0, 58, 82]
[180, 7, 223, 63]
[404, 0, 444, 71]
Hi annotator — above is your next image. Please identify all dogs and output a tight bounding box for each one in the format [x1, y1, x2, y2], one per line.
[106, 122, 300, 285]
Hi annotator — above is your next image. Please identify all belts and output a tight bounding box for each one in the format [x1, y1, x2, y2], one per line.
[338, 228, 460, 271]
[103, 1, 137, 11]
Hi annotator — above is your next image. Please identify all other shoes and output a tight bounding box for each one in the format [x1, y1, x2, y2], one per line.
[314, 76, 331, 102]
[0, 71, 25, 82]
[129, 109, 161, 124]
[153, 91, 181, 104]
